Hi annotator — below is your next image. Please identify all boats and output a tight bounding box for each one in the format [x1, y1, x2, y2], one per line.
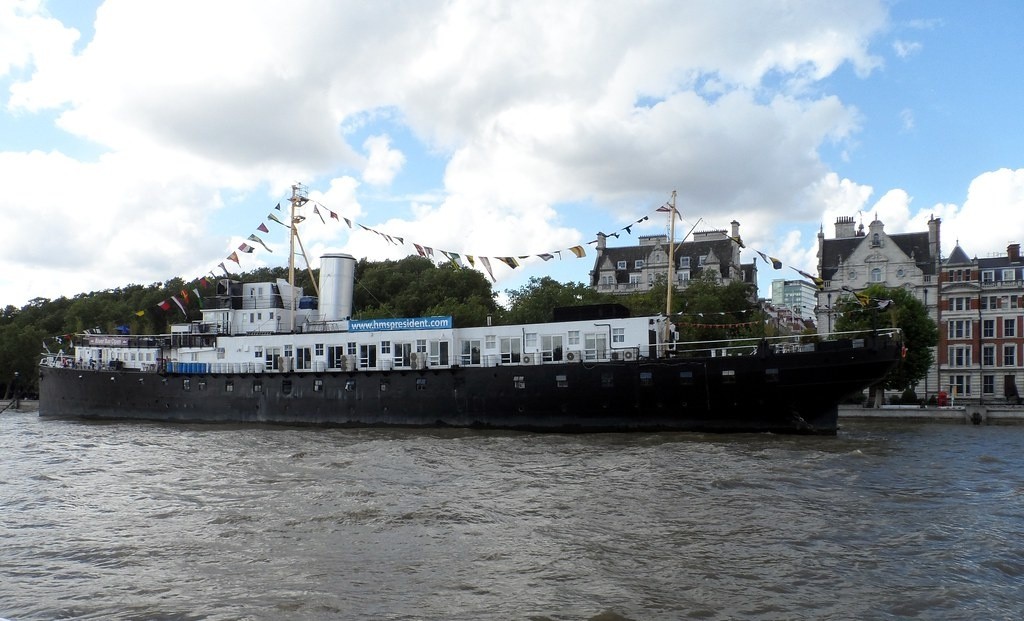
[33, 182, 910, 436]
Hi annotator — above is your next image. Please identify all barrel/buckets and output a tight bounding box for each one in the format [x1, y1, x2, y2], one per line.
[376, 359, 391, 371]
[311, 361, 325, 372]
[167, 362, 263, 373]
[481, 354, 496, 367]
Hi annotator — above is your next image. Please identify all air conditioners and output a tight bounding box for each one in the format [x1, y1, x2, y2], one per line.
[611, 351, 624, 360]
[566, 351, 581, 362]
[278, 357, 290, 372]
[520, 354, 534, 365]
[410, 352, 425, 369]
[340, 354, 354, 371]
[624, 349, 638, 361]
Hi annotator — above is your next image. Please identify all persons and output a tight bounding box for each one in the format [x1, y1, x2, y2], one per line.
[62, 355, 94, 370]
[920, 399, 928, 409]
[164, 356, 170, 372]
[939, 389, 949, 406]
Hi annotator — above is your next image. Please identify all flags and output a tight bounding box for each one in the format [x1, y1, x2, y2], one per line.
[295, 191, 680, 283]
[712, 228, 897, 311]
[41, 197, 287, 354]
